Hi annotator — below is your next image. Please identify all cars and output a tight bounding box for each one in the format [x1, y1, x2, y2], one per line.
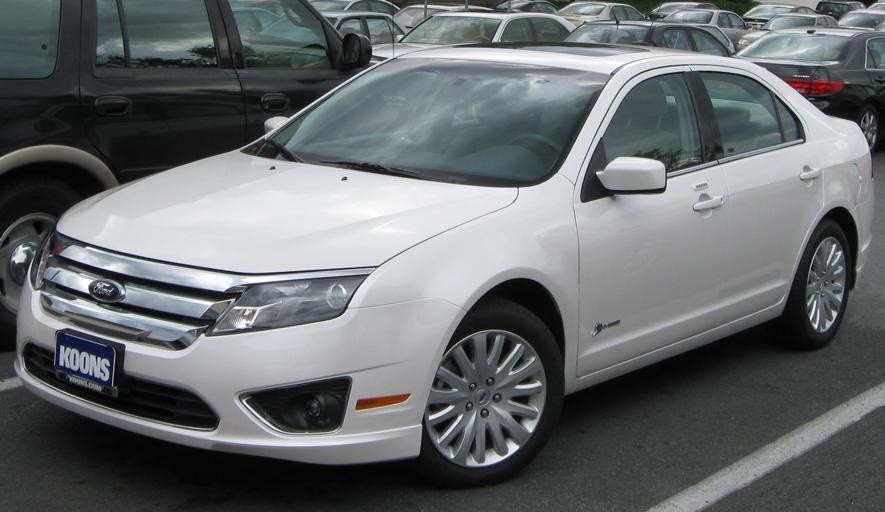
[733, 24, 882, 156]
[15, 42, 874, 483]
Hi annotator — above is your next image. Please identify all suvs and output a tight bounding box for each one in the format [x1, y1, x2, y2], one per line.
[0, 1, 380, 350]
